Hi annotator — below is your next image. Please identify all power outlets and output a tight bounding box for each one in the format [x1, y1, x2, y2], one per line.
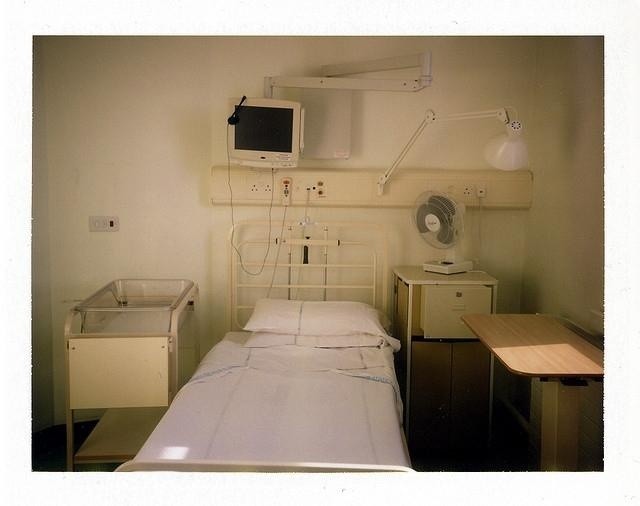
[249, 183, 273, 194]
[461, 185, 474, 197]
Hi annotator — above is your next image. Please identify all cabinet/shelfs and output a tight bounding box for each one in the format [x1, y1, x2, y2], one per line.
[65, 277, 201, 470]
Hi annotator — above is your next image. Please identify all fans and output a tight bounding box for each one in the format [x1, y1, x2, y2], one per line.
[408, 189, 475, 275]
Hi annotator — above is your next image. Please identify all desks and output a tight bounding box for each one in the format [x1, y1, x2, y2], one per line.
[459, 312, 603, 472]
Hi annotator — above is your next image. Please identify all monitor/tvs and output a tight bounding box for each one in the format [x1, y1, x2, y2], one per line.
[226, 96, 305, 168]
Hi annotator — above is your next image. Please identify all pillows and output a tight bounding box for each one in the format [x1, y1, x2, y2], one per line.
[243, 329, 385, 347]
[243, 296, 385, 336]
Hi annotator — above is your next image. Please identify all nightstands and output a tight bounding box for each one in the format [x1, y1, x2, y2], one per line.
[386, 264, 499, 461]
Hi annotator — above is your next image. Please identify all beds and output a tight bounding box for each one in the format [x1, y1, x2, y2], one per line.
[116, 222, 415, 471]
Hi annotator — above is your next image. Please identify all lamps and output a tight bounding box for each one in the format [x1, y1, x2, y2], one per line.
[376, 105, 529, 196]
